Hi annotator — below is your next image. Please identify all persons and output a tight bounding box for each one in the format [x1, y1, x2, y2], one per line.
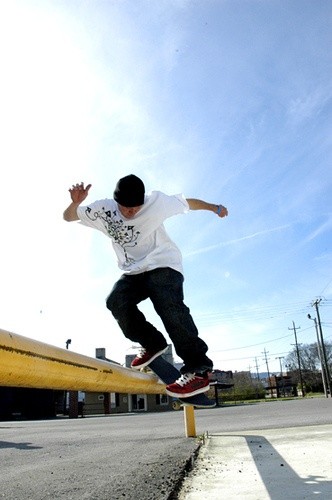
[62, 173, 229, 399]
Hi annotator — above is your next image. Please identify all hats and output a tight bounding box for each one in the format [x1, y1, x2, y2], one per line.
[113, 174, 145, 207]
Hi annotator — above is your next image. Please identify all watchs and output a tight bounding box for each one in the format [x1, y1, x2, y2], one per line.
[215, 204, 222, 214]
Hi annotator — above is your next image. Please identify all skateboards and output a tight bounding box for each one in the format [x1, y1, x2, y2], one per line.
[140, 355, 216, 410]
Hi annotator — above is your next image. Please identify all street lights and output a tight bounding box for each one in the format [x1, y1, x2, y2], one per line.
[66, 339, 71, 349]
[307, 314, 328, 398]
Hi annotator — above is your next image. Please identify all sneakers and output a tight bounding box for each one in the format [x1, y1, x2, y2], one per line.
[165, 371, 210, 398]
[131, 343, 168, 369]
